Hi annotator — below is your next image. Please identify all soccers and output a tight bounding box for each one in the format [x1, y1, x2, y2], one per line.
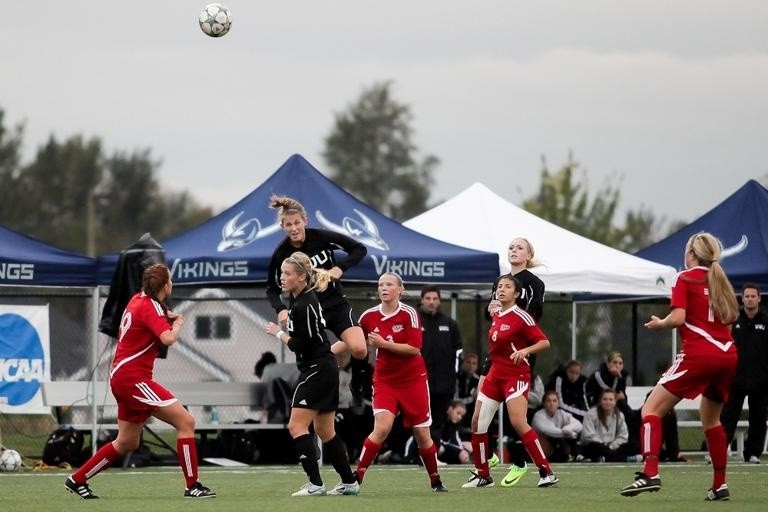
[0, 449, 22, 473]
[199, 3, 232, 38]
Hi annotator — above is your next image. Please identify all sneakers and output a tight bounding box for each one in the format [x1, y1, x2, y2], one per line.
[63, 475, 99, 500]
[626, 454, 644, 463]
[619, 471, 662, 498]
[431, 483, 450, 493]
[704, 483, 731, 503]
[461, 471, 495, 490]
[183, 482, 217, 500]
[749, 456, 761, 464]
[487, 452, 500, 469]
[325, 480, 361, 497]
[501, 461, 528, 488]
[537, 470, 560, 487]
[334, 471, 362, 489]
[291, 482, 327, 498]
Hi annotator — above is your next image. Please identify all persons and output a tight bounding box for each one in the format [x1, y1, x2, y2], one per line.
[504, 352, 686, 461]
[334, 353, 498, 460]
[266, 251, 360, 497]
[330, 271, 448, 492]
[254, 352, 302, 425]
[416, 287, 462, 448]
[267, 197, 368, 365]
[461, 274, 560, 488]
[469, 238, 544, 487]
[621, 232, 739, 499]
[65, 263, 217, 501]
[710, 283, 764, 463]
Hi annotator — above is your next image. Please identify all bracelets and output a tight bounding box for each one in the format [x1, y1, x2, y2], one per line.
[276, 330, 284, 340]
